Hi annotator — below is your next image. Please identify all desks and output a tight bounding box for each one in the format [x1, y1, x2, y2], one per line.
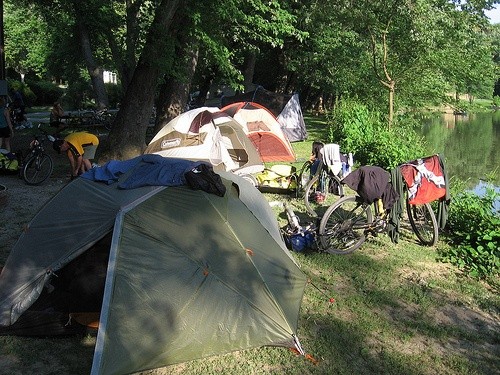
[58, 115, 87, 132]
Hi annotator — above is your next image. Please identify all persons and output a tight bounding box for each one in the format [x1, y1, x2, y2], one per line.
[50, 102, 66, 127]
[0, 99, 14, 153]
[53, 131, 99, 179]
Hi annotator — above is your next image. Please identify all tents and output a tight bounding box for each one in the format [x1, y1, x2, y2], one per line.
[143, 86, 308, 177]
[0, 154, 333, 375]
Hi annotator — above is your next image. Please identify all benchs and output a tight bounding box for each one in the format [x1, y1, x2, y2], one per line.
[78, 123, 105, 135]
[48, 122, 80, 132]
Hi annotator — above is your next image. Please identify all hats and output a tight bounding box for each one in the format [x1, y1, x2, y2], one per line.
[52, 140, 62, 154]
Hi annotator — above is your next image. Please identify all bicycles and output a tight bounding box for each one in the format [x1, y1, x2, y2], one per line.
[19, 124, 57, 185]
[318, 152, 439, 255]
[299, 154, 343, 221]
